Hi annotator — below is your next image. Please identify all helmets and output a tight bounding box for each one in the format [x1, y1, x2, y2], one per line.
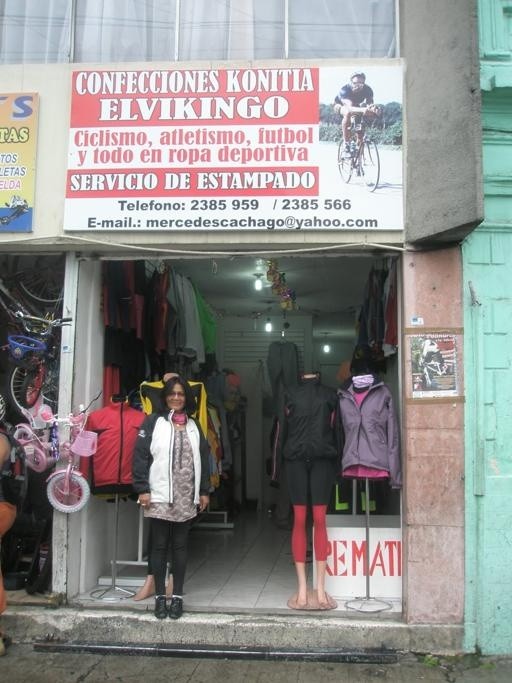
[349, 72, 365, 93]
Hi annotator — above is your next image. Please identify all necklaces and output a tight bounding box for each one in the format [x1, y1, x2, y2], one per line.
[176, 423, 180, 427]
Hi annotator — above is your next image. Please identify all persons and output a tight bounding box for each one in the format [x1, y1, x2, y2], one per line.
[418, 337, 448, 391]
[267, 370, 341, 608]
[0, 417, 28, 614]
[327, 370, 396, 482]
[79, 390, 146, 495]
[331, 71, 384, 178]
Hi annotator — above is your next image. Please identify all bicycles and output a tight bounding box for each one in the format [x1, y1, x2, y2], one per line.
[1, 253, 103, 570]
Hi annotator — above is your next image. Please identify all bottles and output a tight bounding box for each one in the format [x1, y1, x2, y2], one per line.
[351, 139, 356, 152]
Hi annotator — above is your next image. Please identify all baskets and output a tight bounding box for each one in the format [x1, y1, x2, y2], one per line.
[70, 431, 98, 457]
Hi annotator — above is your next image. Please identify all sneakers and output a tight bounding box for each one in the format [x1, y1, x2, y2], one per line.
[155, 597, 167, 618]
[344, 142, 350, 152]
[169, 597, 182, 618]
